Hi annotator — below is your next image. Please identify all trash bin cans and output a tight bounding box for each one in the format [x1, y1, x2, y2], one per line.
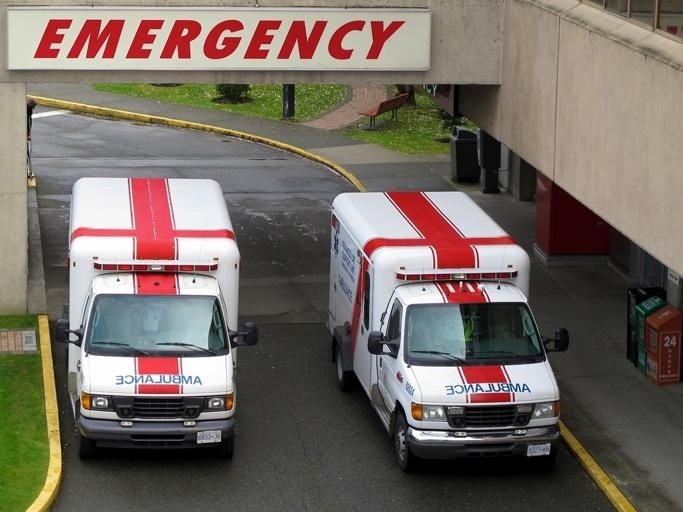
[450, 126, 480, 182]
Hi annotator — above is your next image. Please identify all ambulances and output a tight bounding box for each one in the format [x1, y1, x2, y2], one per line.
[54, 177, 260, 466]
[325, 190, 571, 472]
